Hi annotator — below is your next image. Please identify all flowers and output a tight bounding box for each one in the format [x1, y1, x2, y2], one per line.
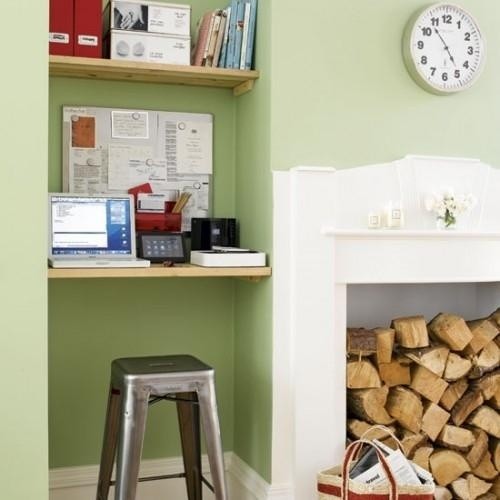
[427, 190, 476, 228]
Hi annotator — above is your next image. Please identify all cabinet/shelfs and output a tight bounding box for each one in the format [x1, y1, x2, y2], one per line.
[48, 55, 272, 283]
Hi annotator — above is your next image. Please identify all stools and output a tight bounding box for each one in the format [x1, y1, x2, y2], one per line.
[96, 354, 228, 500]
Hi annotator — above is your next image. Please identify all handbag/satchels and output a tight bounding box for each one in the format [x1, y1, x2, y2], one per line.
[316, 423, 436, 499]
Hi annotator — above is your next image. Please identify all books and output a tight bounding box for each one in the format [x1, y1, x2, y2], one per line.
[191, 0, 259, 70]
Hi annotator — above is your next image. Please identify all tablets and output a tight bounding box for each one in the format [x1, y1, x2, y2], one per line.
[138, 232, 187, 263]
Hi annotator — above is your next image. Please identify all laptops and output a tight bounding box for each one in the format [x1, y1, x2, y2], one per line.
[49, 192, 151, 268]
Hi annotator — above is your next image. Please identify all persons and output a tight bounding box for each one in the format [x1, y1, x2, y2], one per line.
[113, 3, 148, 31]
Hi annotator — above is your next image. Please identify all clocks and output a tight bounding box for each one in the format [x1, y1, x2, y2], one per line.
[402, 2, 487, 96]
